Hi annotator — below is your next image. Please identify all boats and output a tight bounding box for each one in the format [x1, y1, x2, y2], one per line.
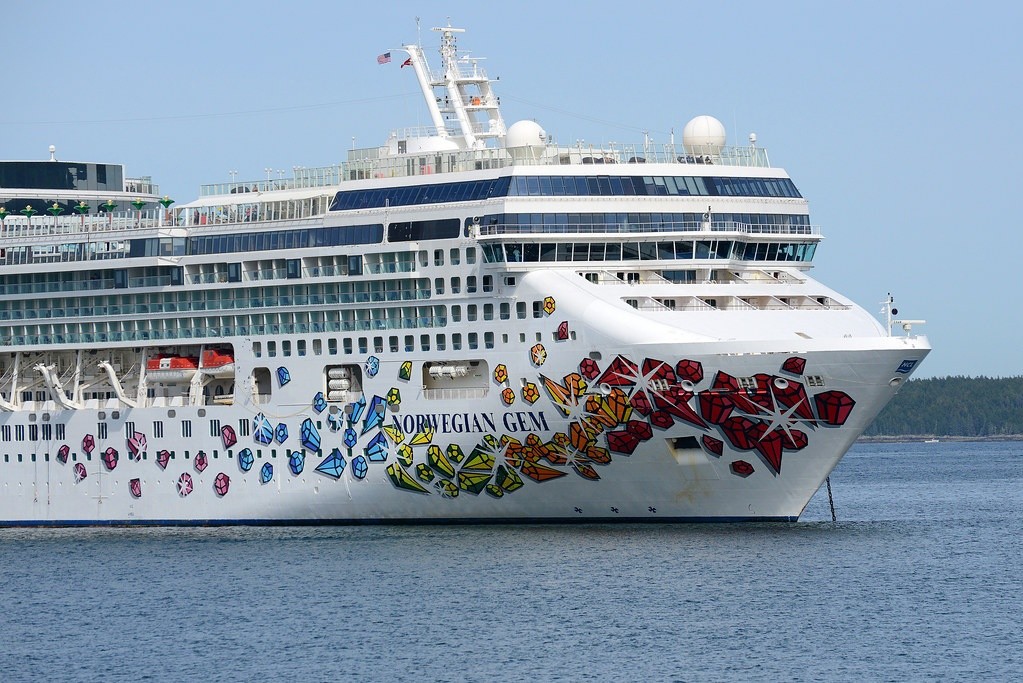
[198, 346, 235, 380]
[146, 351, 200, 385]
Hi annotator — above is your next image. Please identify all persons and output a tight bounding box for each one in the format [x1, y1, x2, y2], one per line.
[169, 204, 258, 226]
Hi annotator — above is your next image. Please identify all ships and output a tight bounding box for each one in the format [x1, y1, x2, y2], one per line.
[0, 15, 934, 527]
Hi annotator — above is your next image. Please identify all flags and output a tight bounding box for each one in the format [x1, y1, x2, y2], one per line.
[377, 52, 391, 64]
